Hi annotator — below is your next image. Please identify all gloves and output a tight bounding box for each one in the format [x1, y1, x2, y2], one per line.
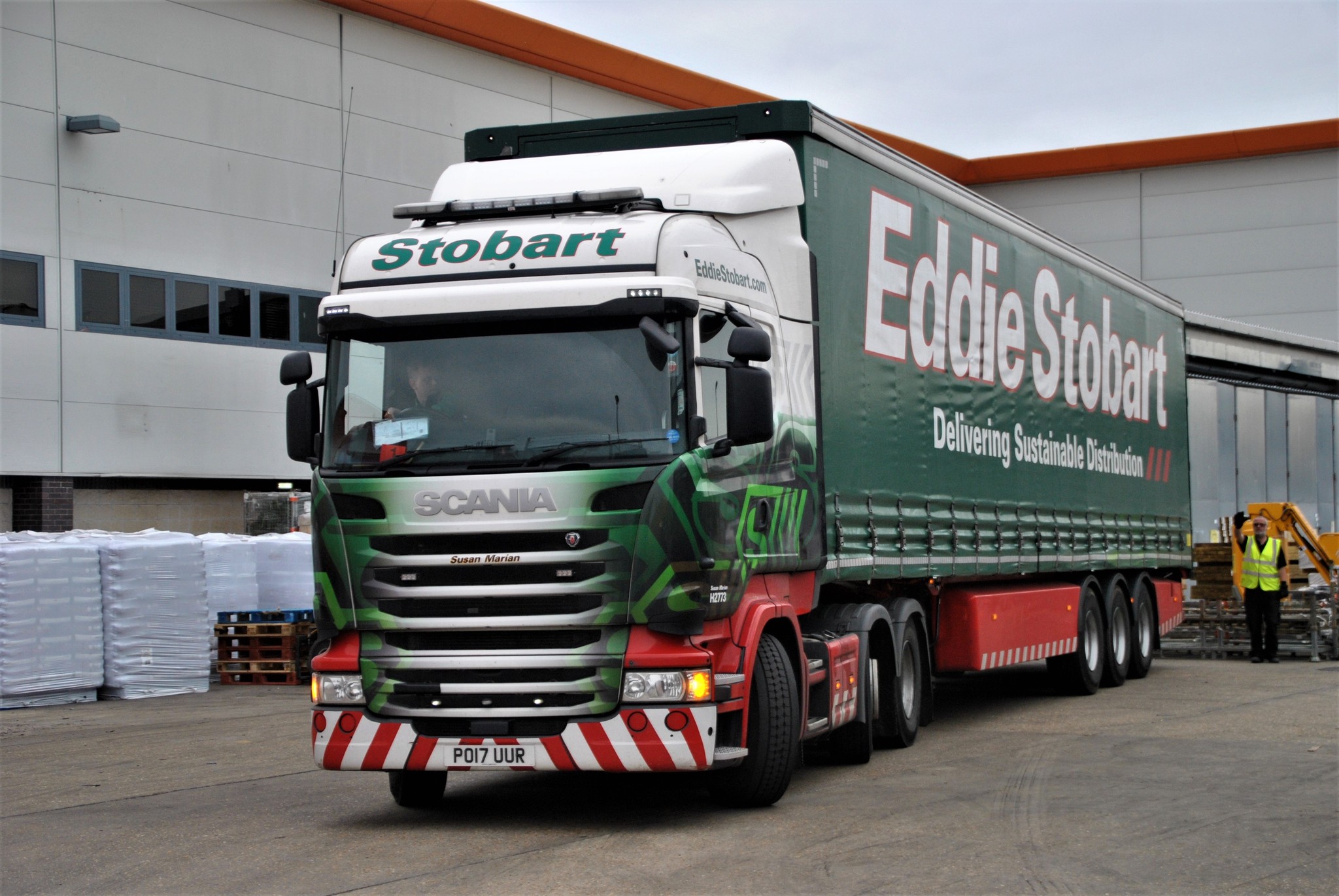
[1279, 581, 1289, 598]
[1233, 511, 1250, 529]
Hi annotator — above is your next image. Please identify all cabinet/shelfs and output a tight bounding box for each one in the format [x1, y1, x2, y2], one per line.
[1159, 586, 1339, 663]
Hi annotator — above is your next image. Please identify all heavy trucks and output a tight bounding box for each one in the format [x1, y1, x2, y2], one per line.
[280, 100, 1202, 805]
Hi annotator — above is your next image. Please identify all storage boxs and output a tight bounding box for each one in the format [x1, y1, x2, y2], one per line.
[216, 648, 298, 661]
[1189, 585, 1235, 600]
[214, 622, 316, 636]
[215, 660, 310, 673]
[1191, 566, 1233, 582]
[218, 636, 300, 648]
[216, 608, 315, 613]
[219, 672, 303, 686]
[1193, 542, 1231, 562]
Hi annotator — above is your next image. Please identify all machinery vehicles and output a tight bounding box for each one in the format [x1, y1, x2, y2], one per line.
[1231, 501, 1339, 607]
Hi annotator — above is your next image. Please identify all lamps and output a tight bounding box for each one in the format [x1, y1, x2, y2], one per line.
[65, 115, 122, 136]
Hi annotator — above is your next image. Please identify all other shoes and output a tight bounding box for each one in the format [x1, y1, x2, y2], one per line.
[1268, 657, 1279, 663]
[1251, 656, 1263, 663]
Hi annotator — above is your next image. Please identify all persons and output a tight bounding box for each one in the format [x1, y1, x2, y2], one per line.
[383, 356, 460, 420]
[1233, 512, 1289, 664]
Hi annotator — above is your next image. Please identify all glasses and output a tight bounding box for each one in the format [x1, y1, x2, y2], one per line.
[1253, 523, 1266, 527]
